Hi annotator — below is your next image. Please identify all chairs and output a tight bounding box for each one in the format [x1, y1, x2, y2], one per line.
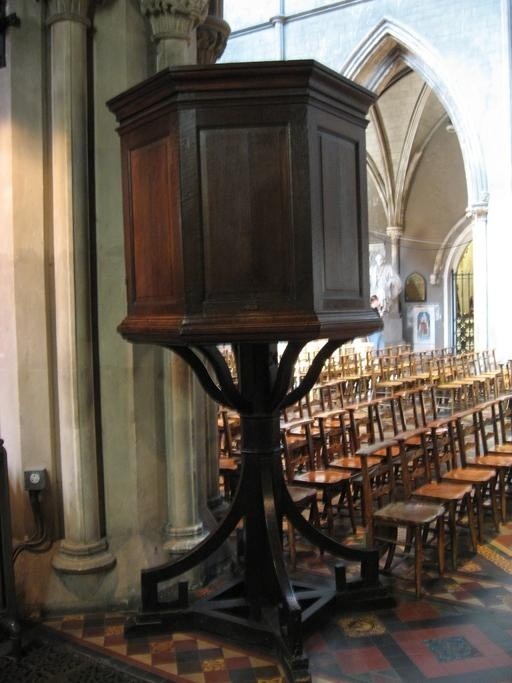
[213, 343, 511, 499]
[282, 419, 356, 545]
[282, 485, 322, 568]
[429, 417, 500, 548]
[358, 439, 446, 598]
[396, 430, 476, 570]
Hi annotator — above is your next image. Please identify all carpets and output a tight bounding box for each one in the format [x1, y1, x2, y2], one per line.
[3, 612, 198, 682]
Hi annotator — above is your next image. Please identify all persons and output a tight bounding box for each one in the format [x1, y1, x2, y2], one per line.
[419, 312, 429, 338]
[366, 296, 384, 359]
